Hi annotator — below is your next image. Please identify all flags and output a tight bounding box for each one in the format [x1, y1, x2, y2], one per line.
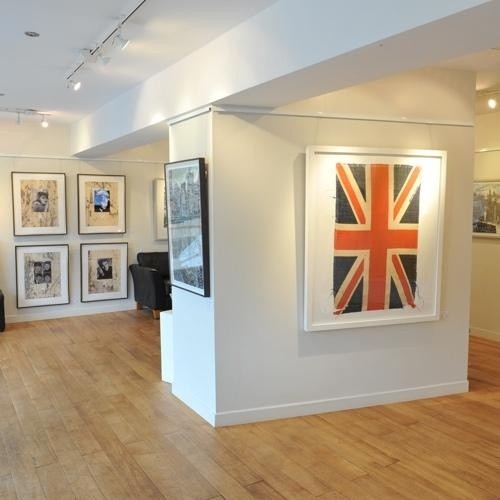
[331, 162, 421, 316]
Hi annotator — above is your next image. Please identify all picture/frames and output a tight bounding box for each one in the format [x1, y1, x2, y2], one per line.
[302, 144, 448, 333]
[162, 157, 210, 298]
[11, 170, 128, 310]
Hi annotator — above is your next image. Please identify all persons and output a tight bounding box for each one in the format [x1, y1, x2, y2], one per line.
[34, 262, 51, 284]
[94, 191, 110, 212]
[96, 258, 112, 279]
[30, 189, 48, 211]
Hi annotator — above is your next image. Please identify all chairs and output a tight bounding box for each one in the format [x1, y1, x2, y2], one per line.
[130, 251, 172, 320]
